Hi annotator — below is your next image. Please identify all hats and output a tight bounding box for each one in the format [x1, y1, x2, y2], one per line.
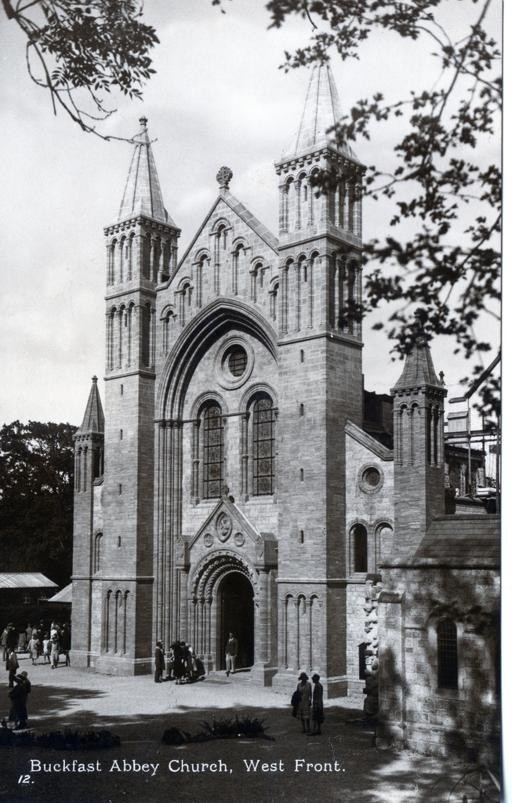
[298, 672, 308, 680]
[12, 674, 25, 683]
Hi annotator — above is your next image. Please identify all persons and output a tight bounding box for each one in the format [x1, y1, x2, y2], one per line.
[306, 674, 324, 737]
[10, 671, 31, 723]
[153, 639, 206, 684]
[296, 671, 313, 733]
[223, 631, 239, 674]
[11, 674, 29, 730]
[1, 618, 71, 669]
[5, 647, 20, 688]
